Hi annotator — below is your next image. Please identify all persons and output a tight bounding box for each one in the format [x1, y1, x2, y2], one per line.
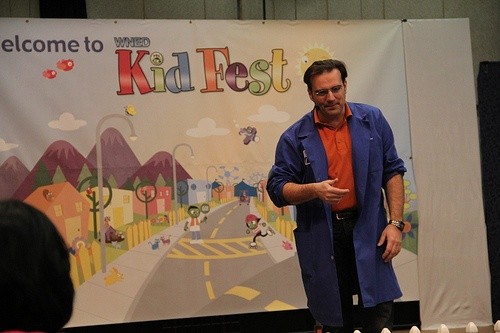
[267, 58, 407, 333]
[0, 199, 73, 333]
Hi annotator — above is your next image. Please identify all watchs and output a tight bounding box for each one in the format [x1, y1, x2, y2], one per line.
[389, 220, 404, 231]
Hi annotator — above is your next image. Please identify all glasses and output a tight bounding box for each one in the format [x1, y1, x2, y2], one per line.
[311, 84, 342, 96]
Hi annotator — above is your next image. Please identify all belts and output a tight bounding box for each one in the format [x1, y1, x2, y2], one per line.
[332, 210, 359, 222]
